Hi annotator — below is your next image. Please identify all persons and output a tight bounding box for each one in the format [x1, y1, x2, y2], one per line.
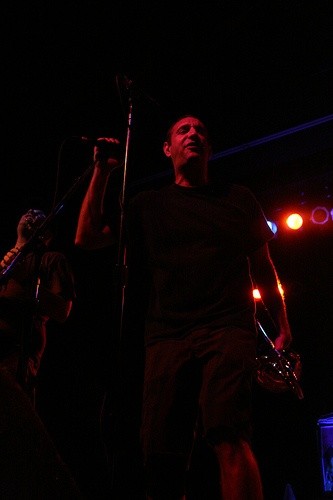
[0, 206, 72, 500]
[75, 114, 293, 500]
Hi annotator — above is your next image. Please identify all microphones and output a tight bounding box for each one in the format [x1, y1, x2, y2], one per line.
[77, 136, 120, 154]
